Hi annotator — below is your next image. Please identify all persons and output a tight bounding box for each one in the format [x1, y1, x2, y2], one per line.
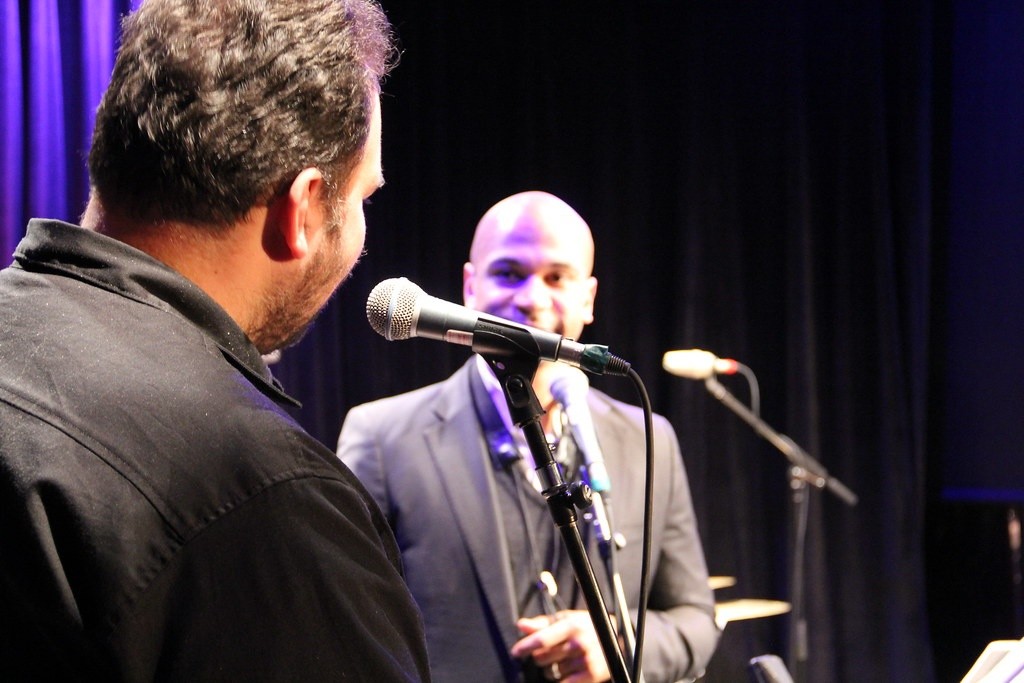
[0, 2, 434, 681]
[334, 187, 722, 683]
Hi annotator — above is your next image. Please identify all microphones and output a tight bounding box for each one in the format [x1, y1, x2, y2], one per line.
[663, 349, 739, 380]
[366, 276, 630, 377]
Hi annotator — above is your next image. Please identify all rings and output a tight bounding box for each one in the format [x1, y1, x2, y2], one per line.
[551, 662, 562, 679]
